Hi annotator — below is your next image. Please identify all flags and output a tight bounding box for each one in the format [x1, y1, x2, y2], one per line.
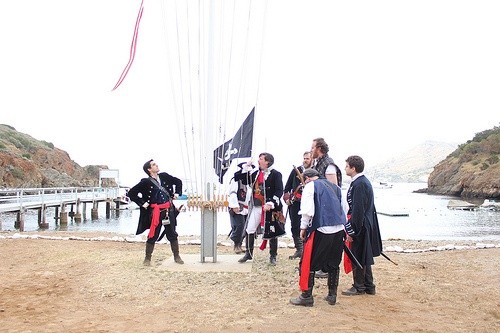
[213, 107, 255, 184]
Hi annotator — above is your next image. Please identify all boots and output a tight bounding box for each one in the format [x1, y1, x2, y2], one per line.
[365, 265, 376, 294]
[289, 234, 304, 258]
[230, 234, 246, 254]
[267, 238, 278, 266]
[342, 264, 367, 295]
[237, 230, 256, 262]
[170, 239, 185, 264]
[288, 270, 315, 307]
[143, 241, 155, 265]
[323, 268, 339, 305]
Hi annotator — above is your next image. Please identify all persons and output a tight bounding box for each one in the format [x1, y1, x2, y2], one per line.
[342, 156, 382, 295]
[129, 159, 184, 265]
[284, 138, 342, 277]
[228, 153, 286, 265]
[289, 169, 346, 305]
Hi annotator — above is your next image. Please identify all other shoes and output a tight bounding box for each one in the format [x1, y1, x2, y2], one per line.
[314, 269, 329, 277]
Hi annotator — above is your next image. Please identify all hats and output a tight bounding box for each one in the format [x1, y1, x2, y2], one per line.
[237, 162, 256, 170]
